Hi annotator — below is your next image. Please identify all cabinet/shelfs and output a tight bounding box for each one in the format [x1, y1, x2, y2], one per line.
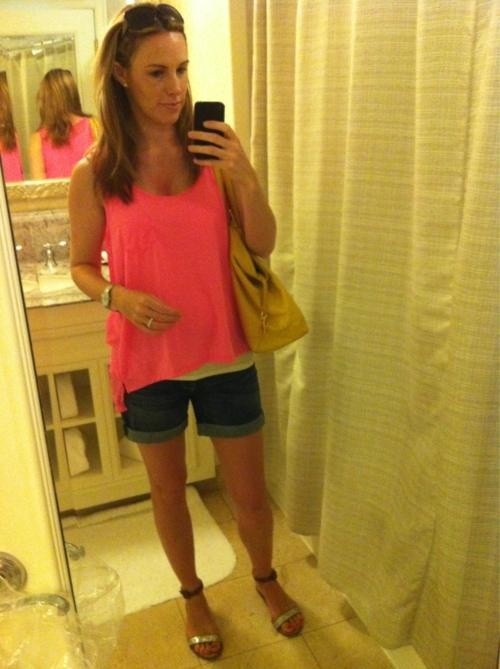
[26, 299, 216, 517]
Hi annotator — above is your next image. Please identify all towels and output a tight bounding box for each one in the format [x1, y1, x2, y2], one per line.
[118, 436, 143, 462]
[56, 374, 78, 420]
[54, 371, 80, 419]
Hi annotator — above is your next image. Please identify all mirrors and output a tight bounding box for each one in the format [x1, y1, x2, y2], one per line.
[0, 0, 108, 211]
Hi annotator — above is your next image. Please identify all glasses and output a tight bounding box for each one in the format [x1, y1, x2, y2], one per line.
[124, 4, 184, 30]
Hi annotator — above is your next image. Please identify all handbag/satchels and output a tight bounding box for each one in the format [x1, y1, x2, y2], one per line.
[215, 167, 310, 354]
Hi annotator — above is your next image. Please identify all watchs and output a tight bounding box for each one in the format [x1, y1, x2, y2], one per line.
[99, 282, 116, 311]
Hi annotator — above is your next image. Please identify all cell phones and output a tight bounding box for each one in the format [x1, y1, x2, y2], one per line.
[194, 102, 224, 159]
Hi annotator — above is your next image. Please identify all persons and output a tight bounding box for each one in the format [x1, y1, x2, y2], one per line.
[64, 0, 307, 664]
[27, 67, 103, 182]
[1, 70, 25, 185]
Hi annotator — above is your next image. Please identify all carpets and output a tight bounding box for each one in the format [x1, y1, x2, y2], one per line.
[60, 485, 238, 625]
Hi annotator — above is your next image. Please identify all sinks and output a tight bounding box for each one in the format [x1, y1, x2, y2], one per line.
[36, 266, 76, 295]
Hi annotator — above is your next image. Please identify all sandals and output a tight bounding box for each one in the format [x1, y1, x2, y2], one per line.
[179, 578, 223, 660]
[254, 567, 303, 638]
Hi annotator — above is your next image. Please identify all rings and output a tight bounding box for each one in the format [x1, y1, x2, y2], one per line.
[146, 317, 155, 329]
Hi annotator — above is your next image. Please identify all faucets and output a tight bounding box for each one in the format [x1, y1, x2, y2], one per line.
[41, 241, 67, 277]
